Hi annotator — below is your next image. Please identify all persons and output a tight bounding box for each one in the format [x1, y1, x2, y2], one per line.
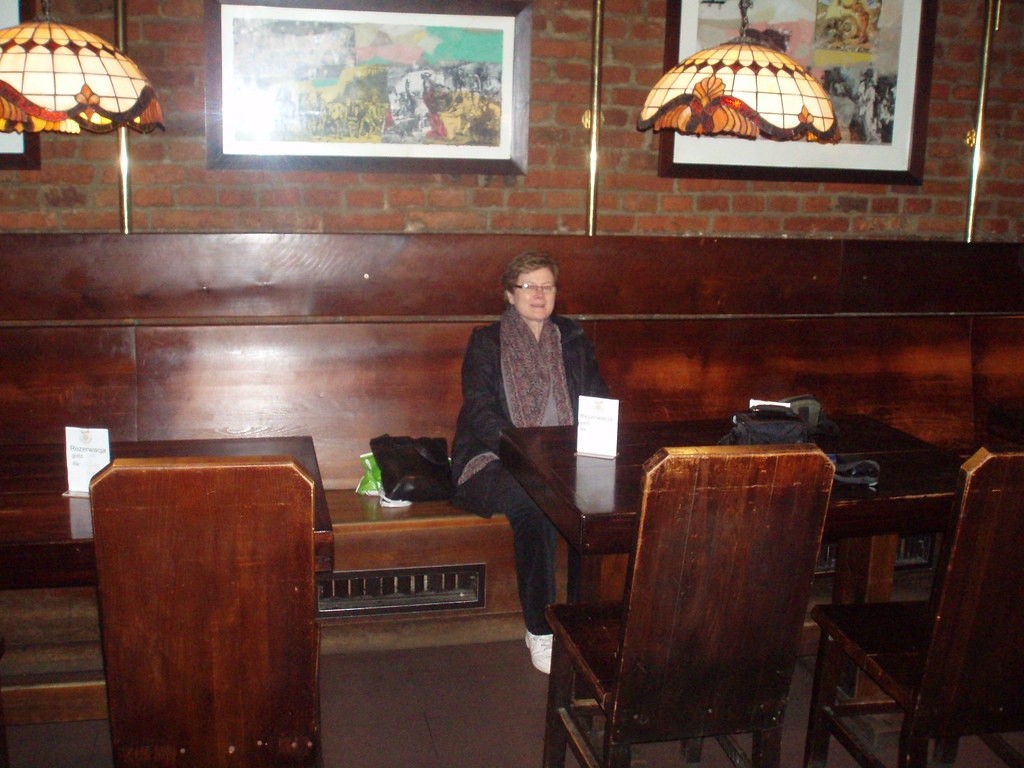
[450, 249, 610, 675]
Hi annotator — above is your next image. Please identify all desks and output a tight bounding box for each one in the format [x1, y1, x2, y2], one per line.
[497, 413, 1024, 768]
[0, 435, 335, 768]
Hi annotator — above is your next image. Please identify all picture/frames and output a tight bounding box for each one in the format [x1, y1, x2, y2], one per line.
[658, 0, 940, 188]
[202, 0, 533, 176]
[0, 0, 42, 171]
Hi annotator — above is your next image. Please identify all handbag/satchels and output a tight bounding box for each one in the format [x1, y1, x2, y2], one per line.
[731, 406, 807, 445]
[779, 394, 821, 430]
[369, 433, 456, 502]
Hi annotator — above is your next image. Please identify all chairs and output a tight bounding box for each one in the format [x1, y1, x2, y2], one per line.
[803, 447, 1024, 768]
[542, 441, 836, 768]
[88, 458, 324, 768]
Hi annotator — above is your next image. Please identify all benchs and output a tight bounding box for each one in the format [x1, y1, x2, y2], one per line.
[0, 234, 1024, 674]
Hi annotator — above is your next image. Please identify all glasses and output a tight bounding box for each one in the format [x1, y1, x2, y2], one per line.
[512, 283, 556, 296]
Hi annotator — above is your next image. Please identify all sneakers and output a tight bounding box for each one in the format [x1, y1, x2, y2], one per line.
[524, 629, 554, 674]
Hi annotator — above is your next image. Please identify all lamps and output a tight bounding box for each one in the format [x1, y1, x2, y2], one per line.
[636, 0, 843, 146]
[0, 0, 167, 135]
